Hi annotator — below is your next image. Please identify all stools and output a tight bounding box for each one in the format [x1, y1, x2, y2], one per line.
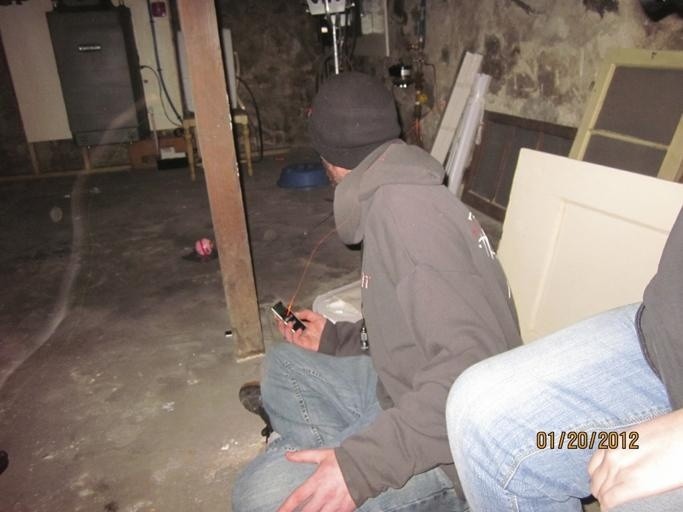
[182, 110, 253, 182]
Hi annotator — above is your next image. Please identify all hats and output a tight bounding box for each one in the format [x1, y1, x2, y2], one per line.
[296, 72, 401, 170]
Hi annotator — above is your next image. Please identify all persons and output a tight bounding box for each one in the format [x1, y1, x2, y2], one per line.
[443, 201, 683, 512]
[230, 71, 526, 512]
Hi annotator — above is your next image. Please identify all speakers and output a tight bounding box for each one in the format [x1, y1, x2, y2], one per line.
[46, 8, 150, 146]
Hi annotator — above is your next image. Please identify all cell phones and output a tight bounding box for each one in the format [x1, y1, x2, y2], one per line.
[270, 301, 305, 334]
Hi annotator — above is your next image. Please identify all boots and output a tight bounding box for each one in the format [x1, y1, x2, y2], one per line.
[239, 381, 274, 444]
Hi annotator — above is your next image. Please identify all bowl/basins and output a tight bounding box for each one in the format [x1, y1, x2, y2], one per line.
[276, 162, 330, 189]
